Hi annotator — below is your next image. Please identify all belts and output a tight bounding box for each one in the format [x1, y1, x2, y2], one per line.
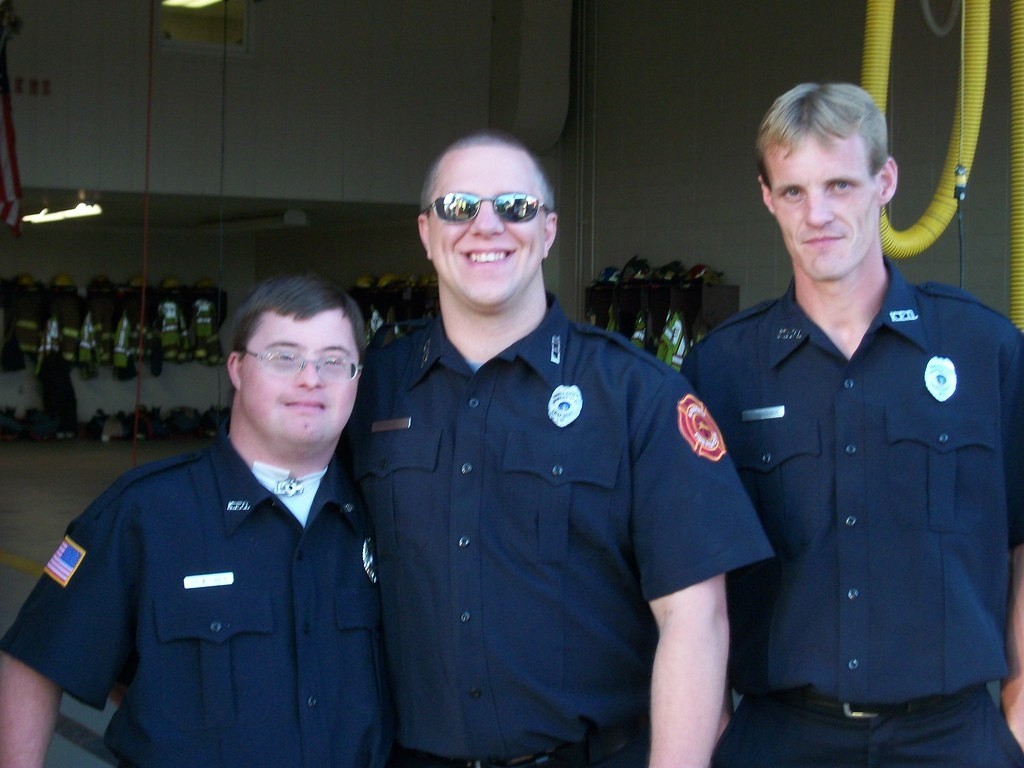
[744, 684, 977, 722]
[396, 714, 647, 768]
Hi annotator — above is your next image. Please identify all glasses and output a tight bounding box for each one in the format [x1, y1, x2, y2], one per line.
[422, 192, 549, 223]
[240, 348, 364, 382]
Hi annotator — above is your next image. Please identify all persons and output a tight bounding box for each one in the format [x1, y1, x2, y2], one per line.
[336, 132, 776, 768]
[677, 83, 1023, 767]
[1, 274, 394, 768]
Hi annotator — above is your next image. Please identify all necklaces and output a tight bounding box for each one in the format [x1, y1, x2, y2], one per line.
[250, 465, 328, 498]
